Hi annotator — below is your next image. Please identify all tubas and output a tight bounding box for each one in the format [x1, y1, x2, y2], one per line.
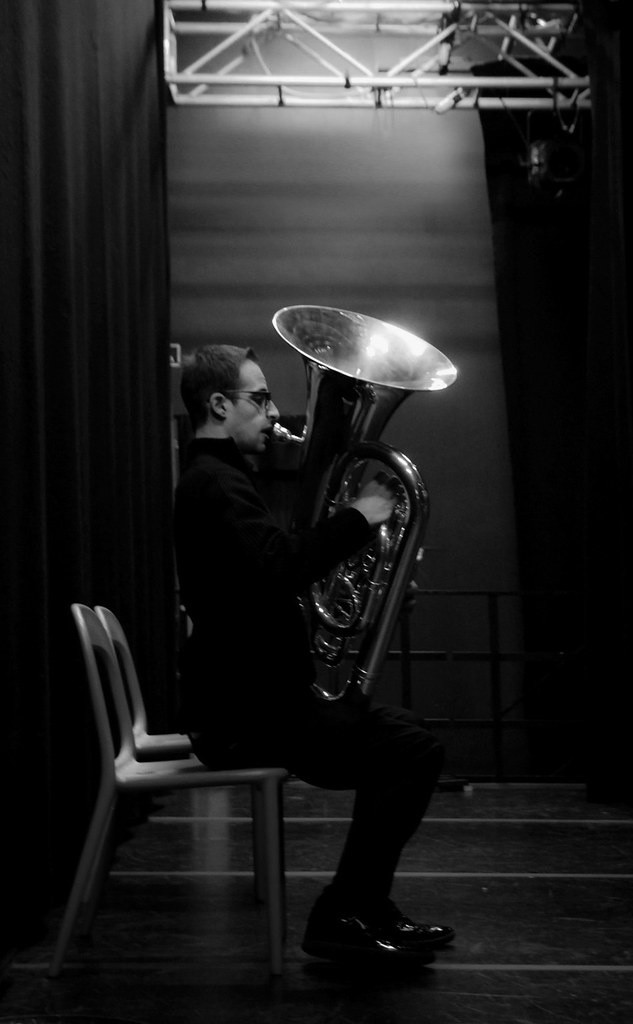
[270, 304, 463, 712]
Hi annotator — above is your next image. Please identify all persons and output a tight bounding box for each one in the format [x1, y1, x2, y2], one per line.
[175, 345, 455, 971]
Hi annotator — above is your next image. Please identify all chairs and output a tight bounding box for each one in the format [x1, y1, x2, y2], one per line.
[40, 602, 291, 995]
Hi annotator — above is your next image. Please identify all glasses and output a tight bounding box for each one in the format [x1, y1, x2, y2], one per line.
[221, 389, 274, 408]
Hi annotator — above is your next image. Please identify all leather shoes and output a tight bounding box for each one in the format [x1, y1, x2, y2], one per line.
[301, 911, 408, 972]
[369, 899, 458, 947]
